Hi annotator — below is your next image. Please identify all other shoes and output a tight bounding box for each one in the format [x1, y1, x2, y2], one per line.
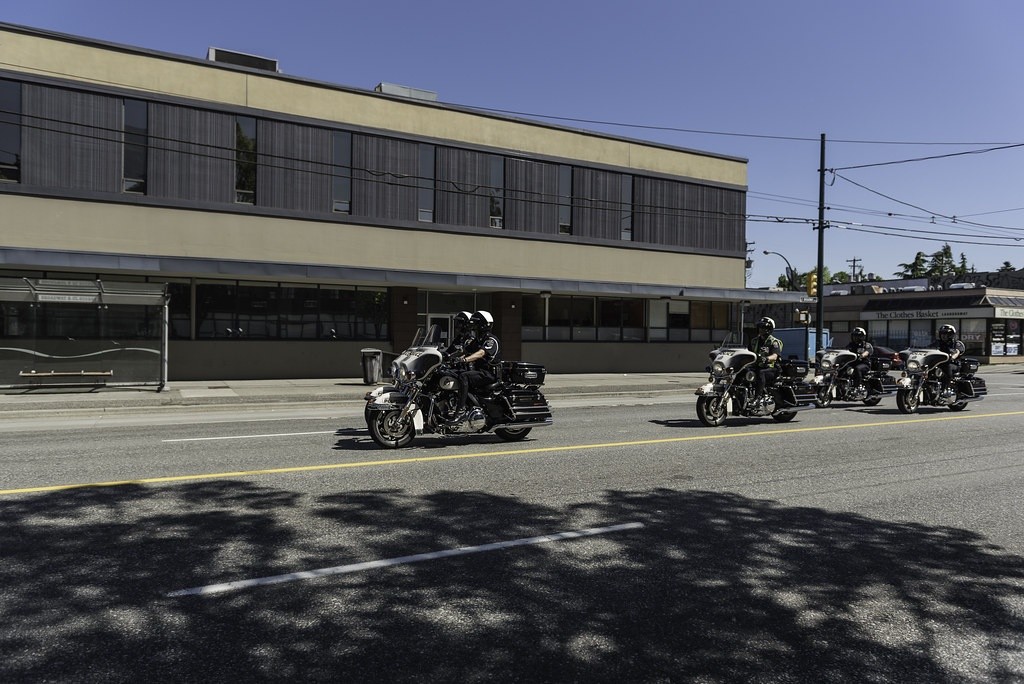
[750, 400, 759, 406]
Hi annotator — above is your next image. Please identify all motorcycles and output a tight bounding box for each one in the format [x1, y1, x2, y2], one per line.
[694, 331, 811, 427]
[806, 336, 888, 409]
[366, 324, 554, 450]
[362, 328, 432, 422]
[891, 337, 981, 415]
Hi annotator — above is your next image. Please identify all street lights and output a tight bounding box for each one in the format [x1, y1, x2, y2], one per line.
[764, 250, 795, 327]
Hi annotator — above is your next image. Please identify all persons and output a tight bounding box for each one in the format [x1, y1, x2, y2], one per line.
[845, 327, 873, 395]
[926, 323, 962, 402]
[444, 310, 498, 424]
[750, 315, 784, 407]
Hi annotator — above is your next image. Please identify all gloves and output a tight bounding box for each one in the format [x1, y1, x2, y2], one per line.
[451, 357, 467, 368]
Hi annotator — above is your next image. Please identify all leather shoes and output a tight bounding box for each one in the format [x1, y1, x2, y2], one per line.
[446, 408, 467, 425]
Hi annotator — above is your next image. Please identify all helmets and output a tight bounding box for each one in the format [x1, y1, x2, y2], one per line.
[939, 324, 956, 334]
[469, 311, 494, 333]
[851, 327, 866, 336]
[454, 311, 472, 334]
[757, 316, 775, 329]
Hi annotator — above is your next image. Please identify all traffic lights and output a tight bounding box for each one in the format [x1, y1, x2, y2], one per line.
[806, 273, 819, 298]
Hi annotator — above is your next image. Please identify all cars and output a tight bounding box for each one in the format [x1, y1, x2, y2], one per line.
[871, 345, 904, 370]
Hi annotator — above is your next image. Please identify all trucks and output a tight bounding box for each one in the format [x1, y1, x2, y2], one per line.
[751, 327, 830, 368]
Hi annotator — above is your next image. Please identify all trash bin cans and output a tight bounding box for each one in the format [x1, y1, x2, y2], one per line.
[360, 347, 381, 385]
